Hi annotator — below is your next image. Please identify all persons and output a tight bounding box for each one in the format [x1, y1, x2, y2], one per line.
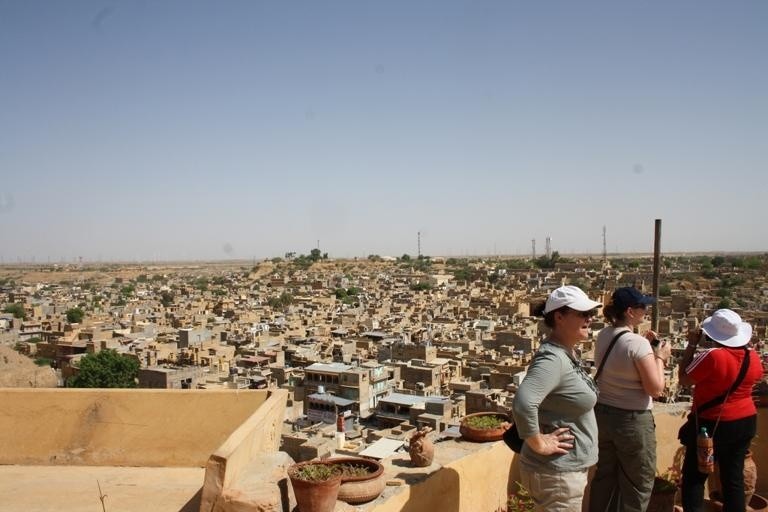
[591, 285, 672, 512]
[677, 307, 764, 511]
[506, 284, 605, 512]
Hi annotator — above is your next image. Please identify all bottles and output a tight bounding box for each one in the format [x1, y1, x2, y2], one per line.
[697, 426, 710, 439]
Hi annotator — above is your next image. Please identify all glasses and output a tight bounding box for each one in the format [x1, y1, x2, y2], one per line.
[633, 304, 646, 309]
[558, 308, 599, 318]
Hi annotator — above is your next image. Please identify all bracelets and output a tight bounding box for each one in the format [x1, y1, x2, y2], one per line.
[655, 355, 665, 363]
[687, 343, 700, 348]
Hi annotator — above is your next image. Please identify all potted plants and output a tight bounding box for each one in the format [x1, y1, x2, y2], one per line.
[649, 476, 678, 512]
[716, 434, 759, 511]
[287, 457, 385, 512]
[459, 411, 514, 443]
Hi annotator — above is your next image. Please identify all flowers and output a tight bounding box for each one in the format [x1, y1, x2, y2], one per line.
[494, 480, 547, 512]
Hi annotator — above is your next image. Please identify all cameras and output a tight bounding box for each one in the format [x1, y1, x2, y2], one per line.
[650, 338, 661, 347]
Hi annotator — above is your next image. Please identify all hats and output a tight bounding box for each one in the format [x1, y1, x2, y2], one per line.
[701, 309, 754, 347]
[609, 285, 657, 311]
[540, 284, 603, 317]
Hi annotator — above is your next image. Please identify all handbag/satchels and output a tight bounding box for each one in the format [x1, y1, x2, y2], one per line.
[503, 423, 524, 453]
[678, 412, 711, 449]
